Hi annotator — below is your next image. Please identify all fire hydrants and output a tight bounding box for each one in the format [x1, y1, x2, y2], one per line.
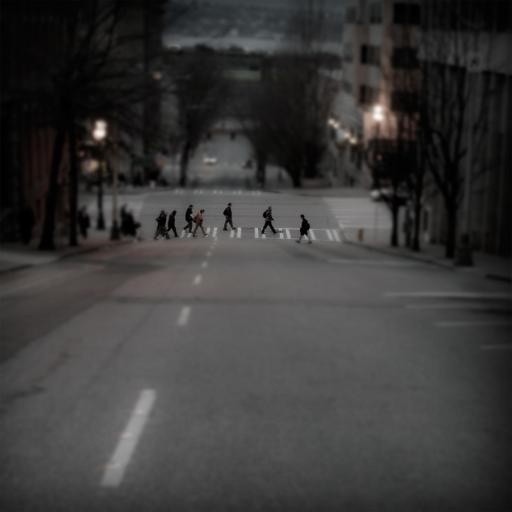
[358, 227, 366, 242]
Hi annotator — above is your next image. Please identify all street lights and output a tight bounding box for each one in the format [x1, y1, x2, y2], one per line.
[92, 117, 109, 231]
[371, 103, 385, 139]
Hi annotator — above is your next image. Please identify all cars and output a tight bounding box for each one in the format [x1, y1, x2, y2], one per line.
[203, 155, 217, 165]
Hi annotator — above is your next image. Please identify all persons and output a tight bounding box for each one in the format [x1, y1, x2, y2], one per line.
[192, 208, 208, 237]
[222, 202, 236, 231]
[401, 209, 412, 247]
[166, 209, 179, 239]
[183, 203, 193, 233]
[260, 205, 276, 234]
[120, 202, 170, 242]
[77, 201, 92, 241]
[296, 213, 313, 244]
